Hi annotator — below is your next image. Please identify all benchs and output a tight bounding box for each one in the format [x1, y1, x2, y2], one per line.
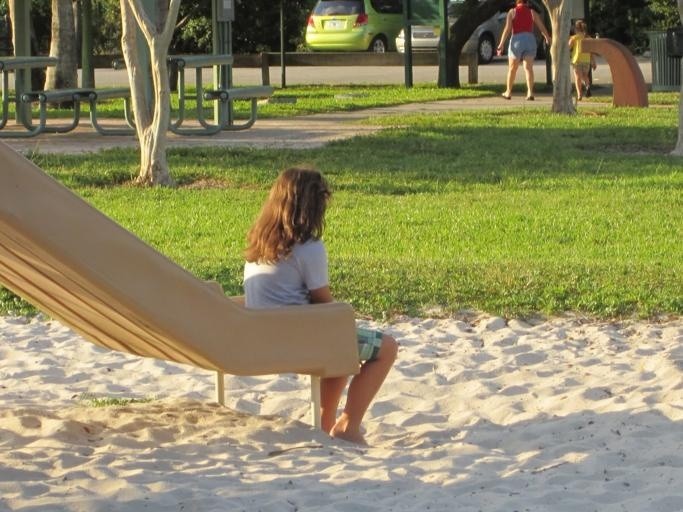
[202, 85, 274, 134]
[19, 87, 129, 138]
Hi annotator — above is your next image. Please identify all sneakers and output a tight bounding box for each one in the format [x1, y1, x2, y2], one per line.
[501, 92, 534, 102]
[577, 86, 591, 103]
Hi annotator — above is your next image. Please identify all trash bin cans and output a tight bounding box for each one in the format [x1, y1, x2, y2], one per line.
[644, 30, 683, 92]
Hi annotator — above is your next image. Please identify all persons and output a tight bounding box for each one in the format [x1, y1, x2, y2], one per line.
[569, 20, 596, 100]
[498, 0, 551, 100]
[244, 168, 397, 445]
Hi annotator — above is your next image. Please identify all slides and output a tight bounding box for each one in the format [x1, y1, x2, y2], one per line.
[0, 137, 361, 379]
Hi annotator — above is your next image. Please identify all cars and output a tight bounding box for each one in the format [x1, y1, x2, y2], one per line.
[305, 0, 549, 64]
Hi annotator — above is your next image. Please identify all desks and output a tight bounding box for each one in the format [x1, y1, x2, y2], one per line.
[0, 55, 57, 138]
[111, 53, 233, 137]
